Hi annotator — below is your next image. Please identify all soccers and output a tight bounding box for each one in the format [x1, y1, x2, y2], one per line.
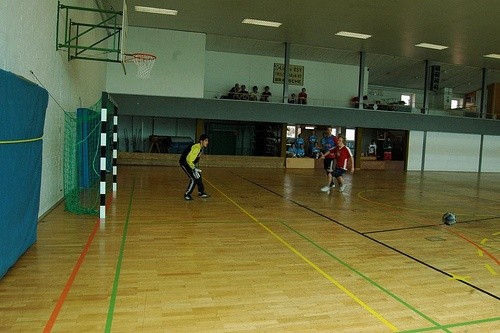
[442, 212, 457, 226]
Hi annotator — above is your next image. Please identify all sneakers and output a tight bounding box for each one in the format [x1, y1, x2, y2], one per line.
[338, 185, 346, 192]
[330, 184, 335, 188]
[198, 193, 208, 197]
[320, 185, 329, 192]
[184, 194, 194, 200]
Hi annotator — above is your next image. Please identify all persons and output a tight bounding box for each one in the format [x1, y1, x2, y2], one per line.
[350, 95, 368, 108]
[318, 136, 354, 192]
[321, 125, 338, 188]
[179, 134, 210, 200]
[228, 82, 307, 105]
[285, 130, 326, 159]
[368, 139, 377, 156]
[382, 136, 393, 161]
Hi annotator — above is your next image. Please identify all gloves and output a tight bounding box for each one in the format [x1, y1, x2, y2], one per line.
[193, 168, 202, 179]
